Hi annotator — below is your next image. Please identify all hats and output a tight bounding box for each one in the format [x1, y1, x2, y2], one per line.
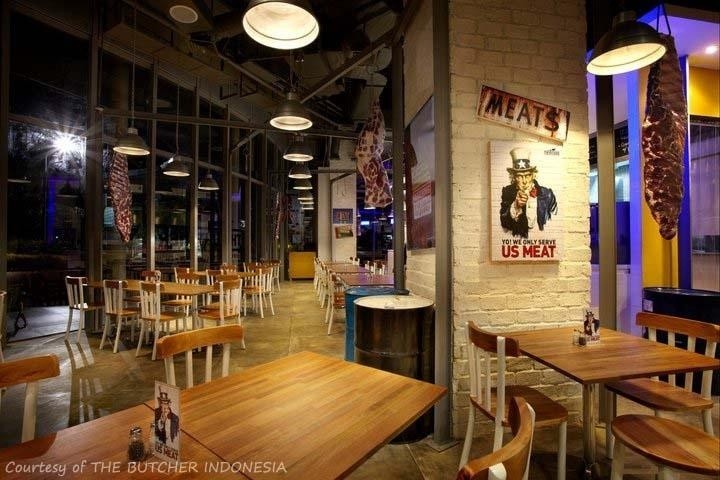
[585, 312, 594, 316]
[157, 392, 170, 401]
[507, 148, 536, 171]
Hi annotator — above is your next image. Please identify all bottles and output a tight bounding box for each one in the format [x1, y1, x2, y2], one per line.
[126, 425, 146, 461]
[572, 329, 587, 345]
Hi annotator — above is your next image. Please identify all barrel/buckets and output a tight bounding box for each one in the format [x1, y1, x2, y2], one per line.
[344, 286, 409, 362]
[353, 294, 435, 445]
[642, 287, 720, 395]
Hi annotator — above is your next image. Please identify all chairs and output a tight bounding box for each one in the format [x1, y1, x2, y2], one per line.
[603, 312, 720, 460]
[64, 259, 281, 361]
[313, 256, 385, 335]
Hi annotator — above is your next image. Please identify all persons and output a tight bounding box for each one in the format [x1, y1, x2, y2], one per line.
[499, 170, 555, 237]
[154, 391, 179, 448]
[583, 306, 599, 338]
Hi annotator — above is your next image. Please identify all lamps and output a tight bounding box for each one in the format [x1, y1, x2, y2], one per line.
[240, 1, 320, 213]
[114, 0, 219, 192]
[586, 9, 669, 77]
